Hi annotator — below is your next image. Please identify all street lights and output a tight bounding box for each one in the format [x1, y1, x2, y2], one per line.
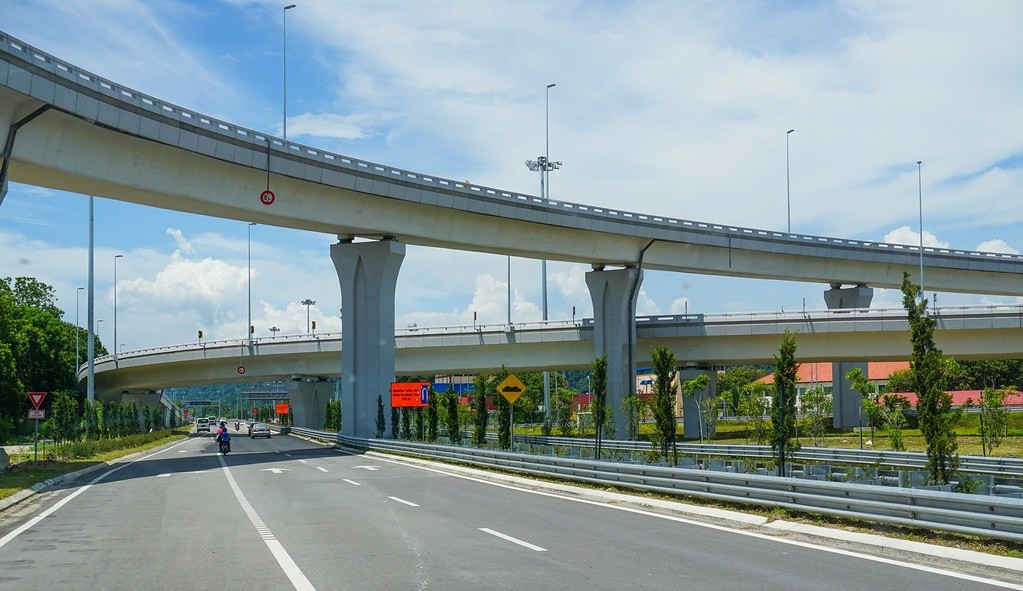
[525, 156, 563, 321]
[120, 342, 126, 354]
[247, 222, 256, 341]
[113, 255, 124, 358]
[544, 82, 558, 202]
[785, 128, 795, 236]
[916, 160, 928, 308]
[96, 319, 105, 349]
[301, 299, 316, 338]
[281, 4, 298, 140]
[268, 326, 281, 338]
[75, 287, 85, 374]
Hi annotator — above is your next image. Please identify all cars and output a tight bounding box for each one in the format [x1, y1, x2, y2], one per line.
[247, 422, 272, 439]
[219, 417, 228, 424]
[215, 418, 220, 422]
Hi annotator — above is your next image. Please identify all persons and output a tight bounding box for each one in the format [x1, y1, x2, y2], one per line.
[235, 419, 240, 430]
[216, 421, 231, 452]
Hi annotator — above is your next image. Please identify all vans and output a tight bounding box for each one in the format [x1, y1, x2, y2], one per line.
[195, 418, 211, 433]
[209, 416, 216, 426]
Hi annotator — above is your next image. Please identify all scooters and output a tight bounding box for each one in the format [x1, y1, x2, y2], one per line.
[235, 425, 239, 431]
[221, 441, 231, 456]
